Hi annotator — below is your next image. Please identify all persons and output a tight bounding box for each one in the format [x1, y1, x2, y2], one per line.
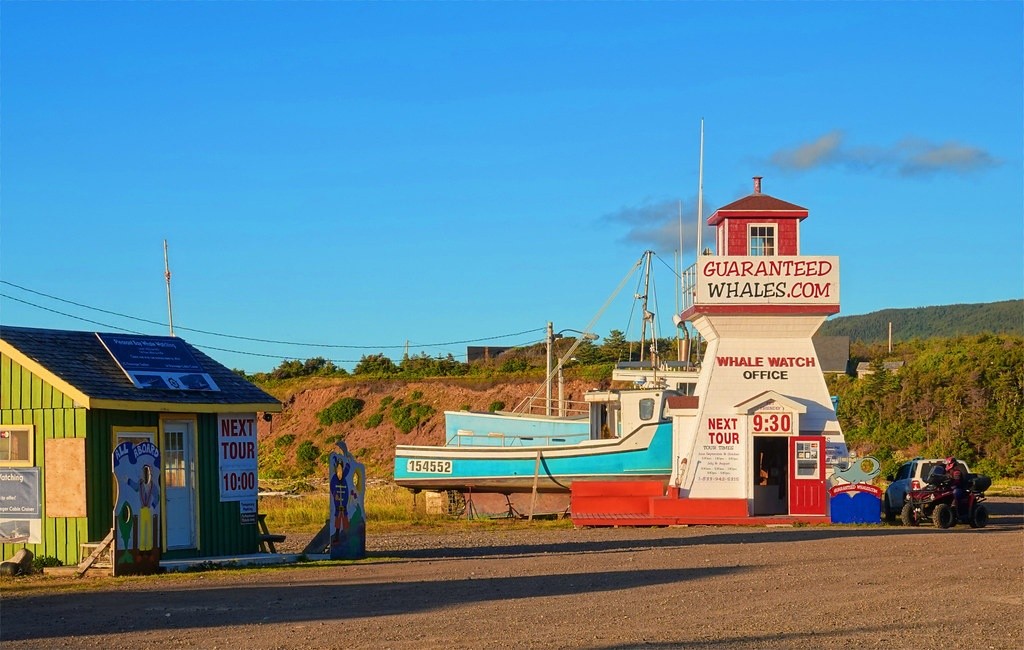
[944, 457, 969, 509]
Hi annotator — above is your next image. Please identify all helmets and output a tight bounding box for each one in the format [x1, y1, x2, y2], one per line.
[945, 457, 957, 471]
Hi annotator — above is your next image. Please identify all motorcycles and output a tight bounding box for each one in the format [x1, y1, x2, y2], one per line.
[899, 473, 992, 531]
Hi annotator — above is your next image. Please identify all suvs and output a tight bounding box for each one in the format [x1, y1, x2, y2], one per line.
[884, 458, 975, 521]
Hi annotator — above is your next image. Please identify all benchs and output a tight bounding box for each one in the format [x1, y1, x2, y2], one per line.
[258, 535, 286, 543]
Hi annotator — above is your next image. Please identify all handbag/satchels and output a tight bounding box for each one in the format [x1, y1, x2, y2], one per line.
[928, 466, 944, 483]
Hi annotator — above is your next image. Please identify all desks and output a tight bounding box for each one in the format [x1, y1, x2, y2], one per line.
[258, 514, 276, 553]
